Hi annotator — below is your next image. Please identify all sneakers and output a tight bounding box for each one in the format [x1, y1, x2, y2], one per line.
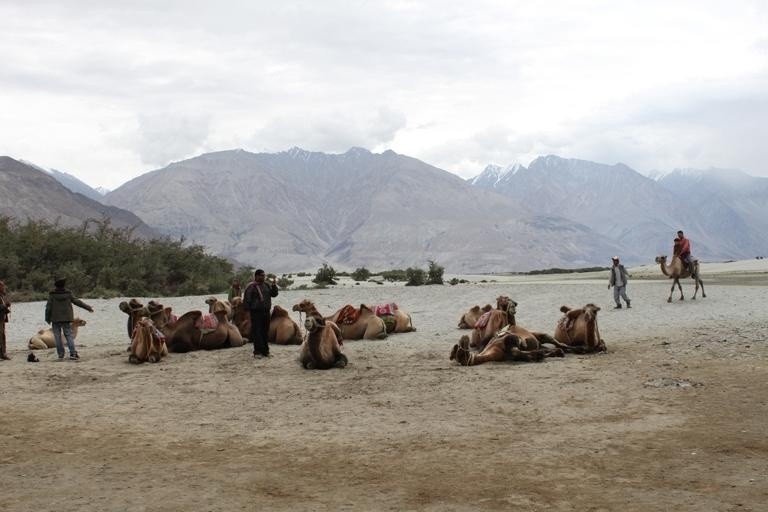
[70, 354, 80, 359]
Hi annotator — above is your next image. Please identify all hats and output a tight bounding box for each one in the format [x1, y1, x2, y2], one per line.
[55, 277, 66, 287]
[612, 256, 620, 260]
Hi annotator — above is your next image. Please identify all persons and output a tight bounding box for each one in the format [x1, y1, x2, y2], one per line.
[44, 277, 94, 360]
[243, 269, 278, 358]
[227, 279, 242, 303]
[674, 238, 689, 272]
[673, 230, 697, 279]
[0, 281, 12, 360]
[607, 255, 632, 309]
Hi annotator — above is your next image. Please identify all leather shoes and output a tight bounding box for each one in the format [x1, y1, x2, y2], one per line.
[614, 300, 632, 308]
